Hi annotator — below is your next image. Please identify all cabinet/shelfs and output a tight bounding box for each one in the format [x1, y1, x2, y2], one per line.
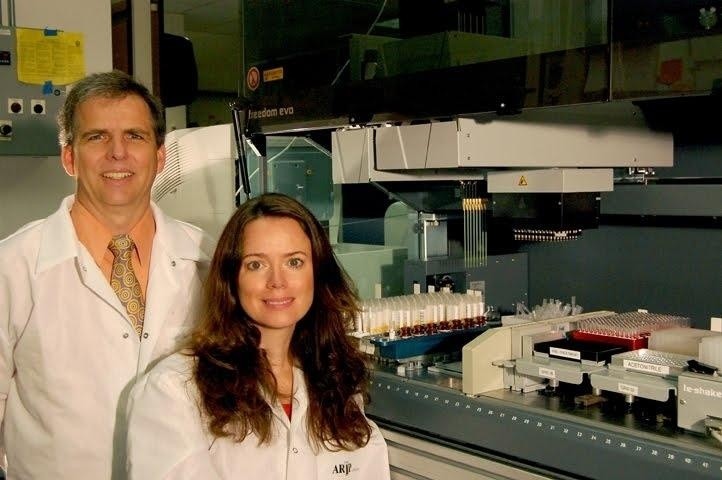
[354, 329, 721, 478]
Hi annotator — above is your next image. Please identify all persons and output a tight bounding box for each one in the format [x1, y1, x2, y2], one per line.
[0, 69, 219, 479]
[125, 193, 393, 479]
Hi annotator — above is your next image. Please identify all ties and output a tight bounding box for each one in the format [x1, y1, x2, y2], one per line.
[108, 231, 146, 343]
